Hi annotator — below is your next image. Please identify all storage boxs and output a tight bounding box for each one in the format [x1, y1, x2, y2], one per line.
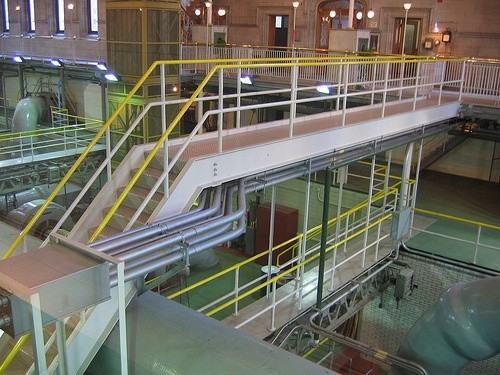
[254, 202, 299, 270]
